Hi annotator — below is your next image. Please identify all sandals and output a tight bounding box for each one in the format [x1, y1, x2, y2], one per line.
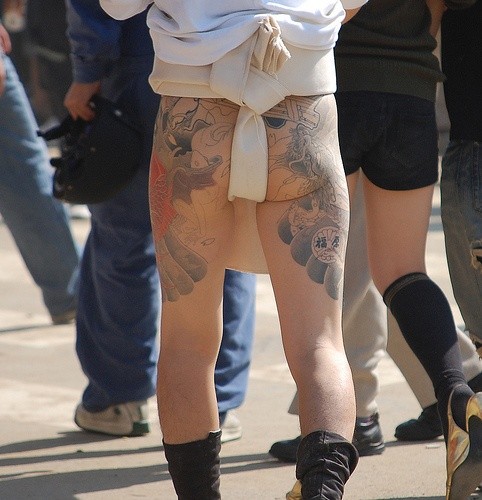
[445, 382, 482, 499]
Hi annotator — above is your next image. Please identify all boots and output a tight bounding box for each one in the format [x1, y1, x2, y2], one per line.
[160, 425, 224, 500]
[295, 432, 359, 500]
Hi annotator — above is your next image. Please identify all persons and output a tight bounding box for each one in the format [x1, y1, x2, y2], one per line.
[0, 0, 257, 443]
[268, 0, 482, 500]
[100, 0, 369, 500]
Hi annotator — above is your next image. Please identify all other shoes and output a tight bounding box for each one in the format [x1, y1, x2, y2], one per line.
[74, 396, 149, 436]
[392, 371, 482, 442]
[218, 413, 242, 442]
[268, 413, 385, 463]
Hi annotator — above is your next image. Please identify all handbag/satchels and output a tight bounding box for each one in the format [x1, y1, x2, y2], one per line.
[36, 92, 146, 205]
